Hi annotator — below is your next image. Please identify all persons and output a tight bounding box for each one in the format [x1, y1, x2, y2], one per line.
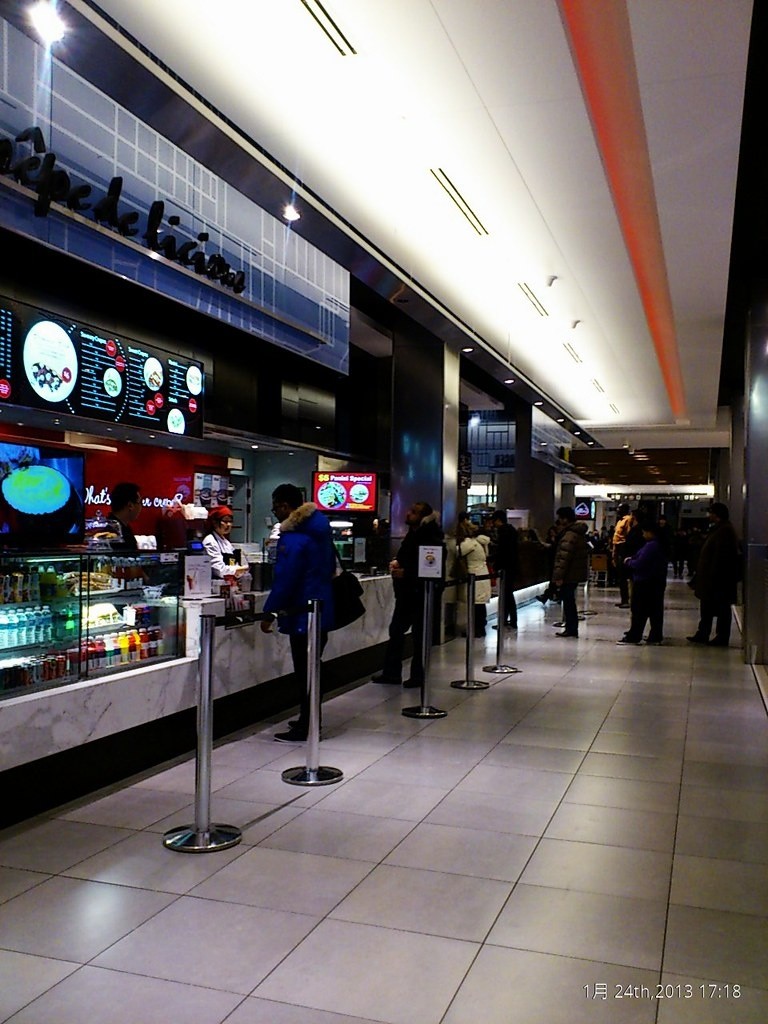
[262, 483, 338, 743]
[619, 520, 668, 644]
[455, 508, 520, 638]
[200, 505, 250, 580]
[97, 480, 153, 586]
[550, 505, 589, 637]
[686, 501, 738, 647]
[369, 500, 444, 689]
[535, 501, 712, 609]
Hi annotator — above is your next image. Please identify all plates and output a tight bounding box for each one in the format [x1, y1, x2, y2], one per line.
[103, 368, 122, 397]
[144, 358, 164, 391]
[349, 484, 369, 503]
[167, 409, 185, 433]
[318, 482, 346, 509]
[186, 366, 202, 395]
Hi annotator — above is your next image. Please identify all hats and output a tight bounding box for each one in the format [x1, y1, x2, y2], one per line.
[208, 504, 232, 517]
[705, 502, 730, 515]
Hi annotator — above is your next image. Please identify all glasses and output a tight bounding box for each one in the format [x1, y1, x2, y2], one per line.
[270, 506, 282, 514]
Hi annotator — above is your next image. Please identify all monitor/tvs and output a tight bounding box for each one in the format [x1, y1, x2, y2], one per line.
[311, 471, 380, 520]
[0, 442, 85, 550]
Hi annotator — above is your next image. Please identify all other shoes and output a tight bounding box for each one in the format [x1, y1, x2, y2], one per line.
[614, 639, 642, 645]
[535, 594, 548, 604]
[492, 624, 516, 631]
[641, 635, 664, 646]
[403, 678, 420, 687]
[273, 730, 323, 745]
[288, 720, 300, 728]
[370, 673, 403, 684]
[555, 629, 579, 637]
[686, 636, 709, 644]
[710, 640, 728, 646]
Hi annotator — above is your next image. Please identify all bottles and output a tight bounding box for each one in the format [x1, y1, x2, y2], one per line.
[242, 571, 251, 592]
[66, 626, 163, 676]
[17, 609, 27, 646]
[96, 557, 144, 591]
[42, 605, 51, 641]
[0, 611, 8, 649]
[8, 608, 18, 647]
[0, 564, 66, 602]
[25, 608, 35, 644]
[34, 607, 43, 643]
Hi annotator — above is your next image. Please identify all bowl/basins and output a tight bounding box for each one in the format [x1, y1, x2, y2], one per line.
[0, 466, 80, 537]
[425, 555, 436, 566]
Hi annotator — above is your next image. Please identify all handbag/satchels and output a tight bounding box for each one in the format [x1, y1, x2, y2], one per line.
[328, 542, 367, 631]
